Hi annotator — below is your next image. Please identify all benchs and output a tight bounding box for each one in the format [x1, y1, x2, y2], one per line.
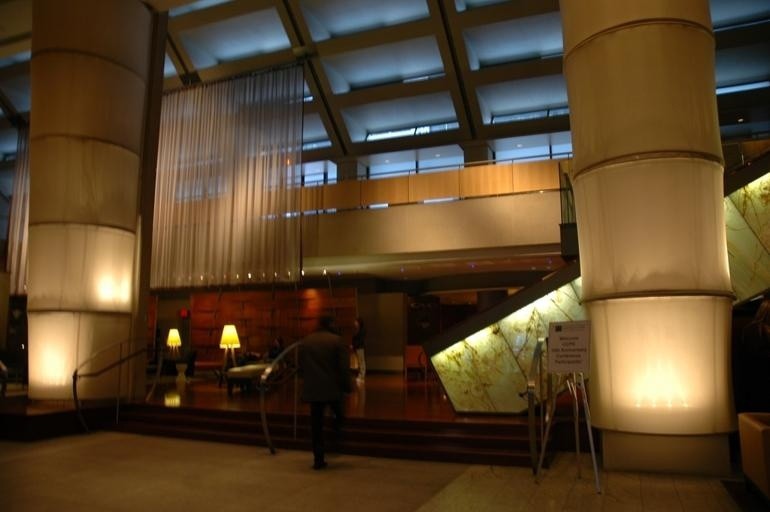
[227, 363, 281, 397]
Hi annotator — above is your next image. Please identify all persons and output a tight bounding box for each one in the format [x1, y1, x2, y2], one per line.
[295, 315, 353, 469]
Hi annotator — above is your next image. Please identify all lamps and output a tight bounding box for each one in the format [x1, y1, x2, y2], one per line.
[218, 324, 241, 373]
[166, 328, 182, 360]
